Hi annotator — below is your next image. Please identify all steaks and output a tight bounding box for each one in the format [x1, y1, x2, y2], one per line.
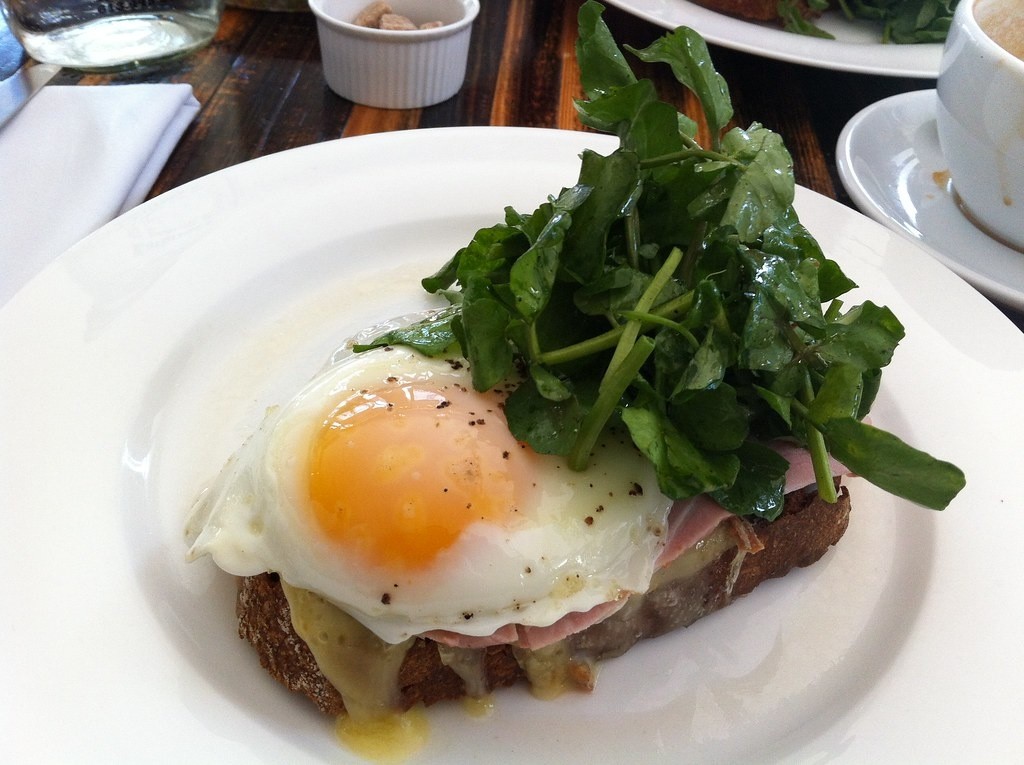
[235, 470, 855, 716]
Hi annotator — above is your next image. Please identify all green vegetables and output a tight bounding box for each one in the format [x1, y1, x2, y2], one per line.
[348, 0, 966, 522]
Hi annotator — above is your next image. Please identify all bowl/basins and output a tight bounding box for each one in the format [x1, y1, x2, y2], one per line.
[310, 0, 481, 109]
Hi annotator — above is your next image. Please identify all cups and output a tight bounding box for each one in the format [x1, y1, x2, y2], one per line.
[933, 0, 1024, 252]
[0, 0, 219, 74]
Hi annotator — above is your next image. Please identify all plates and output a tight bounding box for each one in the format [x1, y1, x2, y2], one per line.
[835, 88, 1024, 310]
[607, 0, 942, 78]
[1, 127, 1024, 765]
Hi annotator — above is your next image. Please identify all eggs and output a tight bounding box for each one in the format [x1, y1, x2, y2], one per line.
[182, 301, 675, 644]
